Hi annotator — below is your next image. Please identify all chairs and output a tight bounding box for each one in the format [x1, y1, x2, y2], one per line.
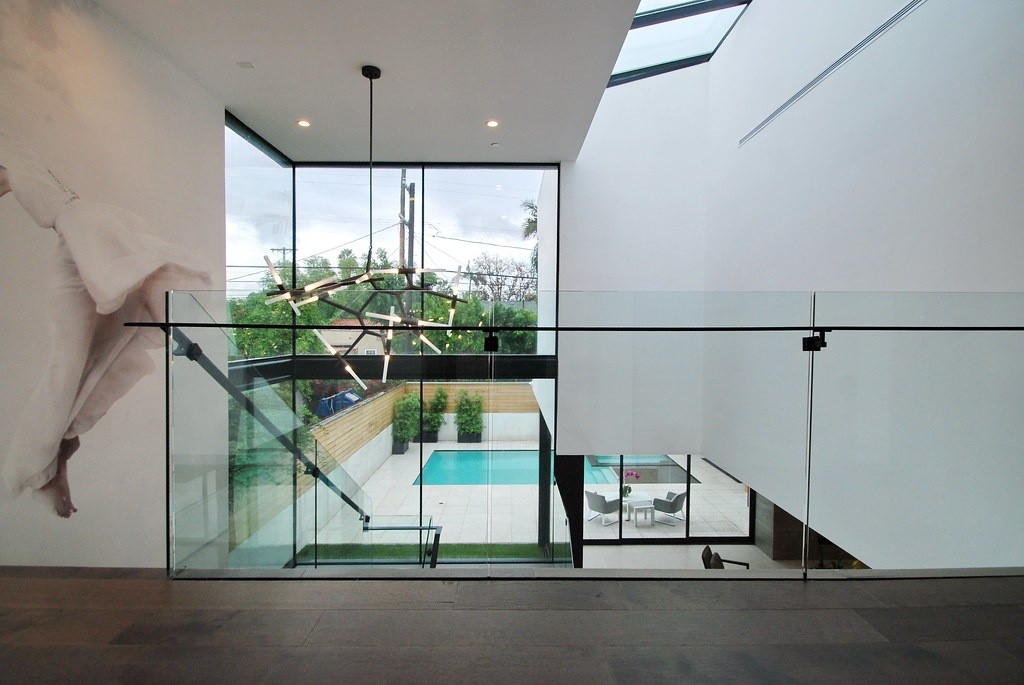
[585, 490, 620, 526]
[701, 545, 749, 569]
[653, 491, 686, 526]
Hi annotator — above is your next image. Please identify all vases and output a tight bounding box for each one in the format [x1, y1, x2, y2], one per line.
[623, 485, 632, 497]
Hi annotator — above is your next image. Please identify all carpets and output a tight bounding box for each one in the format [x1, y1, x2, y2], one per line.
[282, 542, 572, 569]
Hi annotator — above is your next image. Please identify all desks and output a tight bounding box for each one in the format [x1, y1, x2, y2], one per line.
[625, 501, 655, 528]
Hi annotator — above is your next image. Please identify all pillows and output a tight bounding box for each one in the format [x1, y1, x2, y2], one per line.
[711, 552, 724, 569]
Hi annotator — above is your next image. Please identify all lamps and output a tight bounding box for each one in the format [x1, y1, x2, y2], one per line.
[260, 66, 469, 390]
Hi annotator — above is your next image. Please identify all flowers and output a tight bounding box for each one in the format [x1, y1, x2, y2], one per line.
[624, 471, 641, 485]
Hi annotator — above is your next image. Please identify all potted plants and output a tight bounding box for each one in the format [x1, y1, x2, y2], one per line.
[453, 388, 486, 443]
[391, 386, 448, 454]
